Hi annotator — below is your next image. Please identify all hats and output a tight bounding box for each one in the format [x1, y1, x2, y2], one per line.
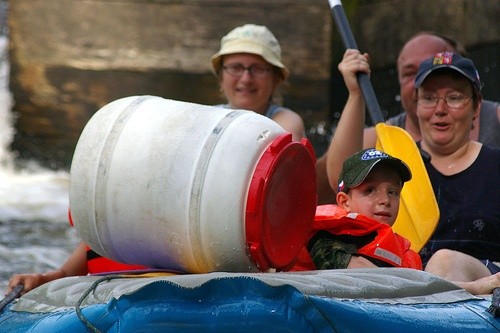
[211, 23, 289, 83]
[337, 147, 411, 194]
[413, 52, 481, 90]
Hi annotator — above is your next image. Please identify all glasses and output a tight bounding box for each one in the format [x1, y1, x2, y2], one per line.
[417, 93, 472, 109]
[222, 62, 273, 79]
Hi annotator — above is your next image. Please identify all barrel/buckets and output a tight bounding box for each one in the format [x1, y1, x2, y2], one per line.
[69, 95, 318, 274]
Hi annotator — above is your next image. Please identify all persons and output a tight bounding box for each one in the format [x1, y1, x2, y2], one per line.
[306, 32, 500, 295]
[9, 210, 147, 297]
[211, 24, 307, 142]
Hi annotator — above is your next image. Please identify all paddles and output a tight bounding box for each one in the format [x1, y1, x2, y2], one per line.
[328, 0, 441, 254]
[0, 285, 24, 313]
